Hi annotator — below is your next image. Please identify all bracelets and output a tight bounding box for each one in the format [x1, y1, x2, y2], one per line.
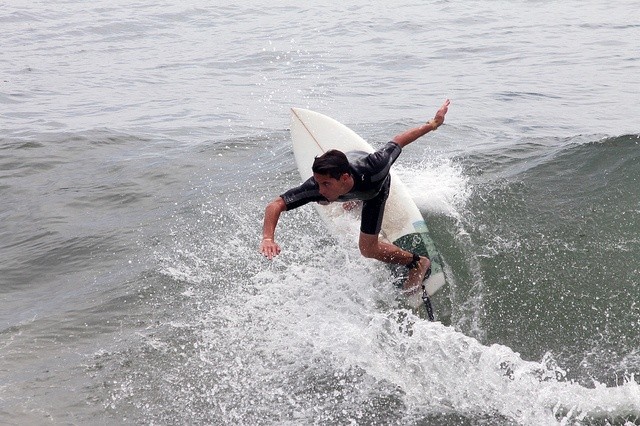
[427, 118, 439, 132]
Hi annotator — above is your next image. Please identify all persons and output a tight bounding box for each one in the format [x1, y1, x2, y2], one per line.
[260, 100, 451, 295]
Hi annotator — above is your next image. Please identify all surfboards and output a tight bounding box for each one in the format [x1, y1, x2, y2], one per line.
[290, 107, 451, 325]
